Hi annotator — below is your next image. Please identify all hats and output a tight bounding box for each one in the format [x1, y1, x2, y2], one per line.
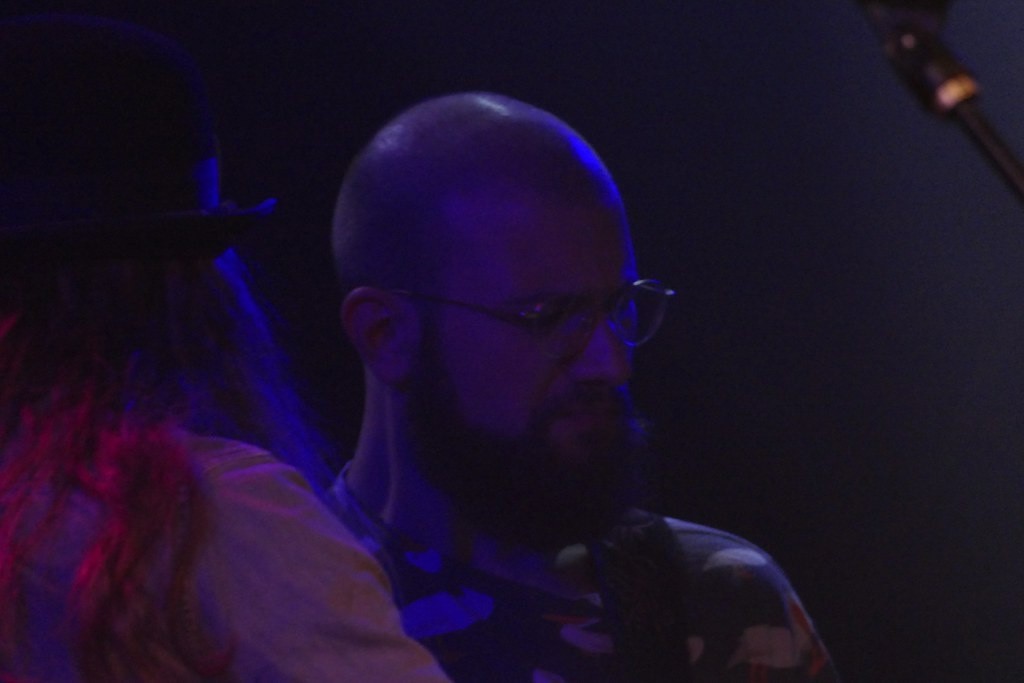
[0, 12, 276, 256]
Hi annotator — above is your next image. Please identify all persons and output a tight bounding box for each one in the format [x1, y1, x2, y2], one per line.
[0, 13, 450, 683]
[331, 93, 837, 683]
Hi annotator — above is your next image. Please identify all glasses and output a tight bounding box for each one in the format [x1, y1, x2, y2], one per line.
[388, 278, 679, 358]
